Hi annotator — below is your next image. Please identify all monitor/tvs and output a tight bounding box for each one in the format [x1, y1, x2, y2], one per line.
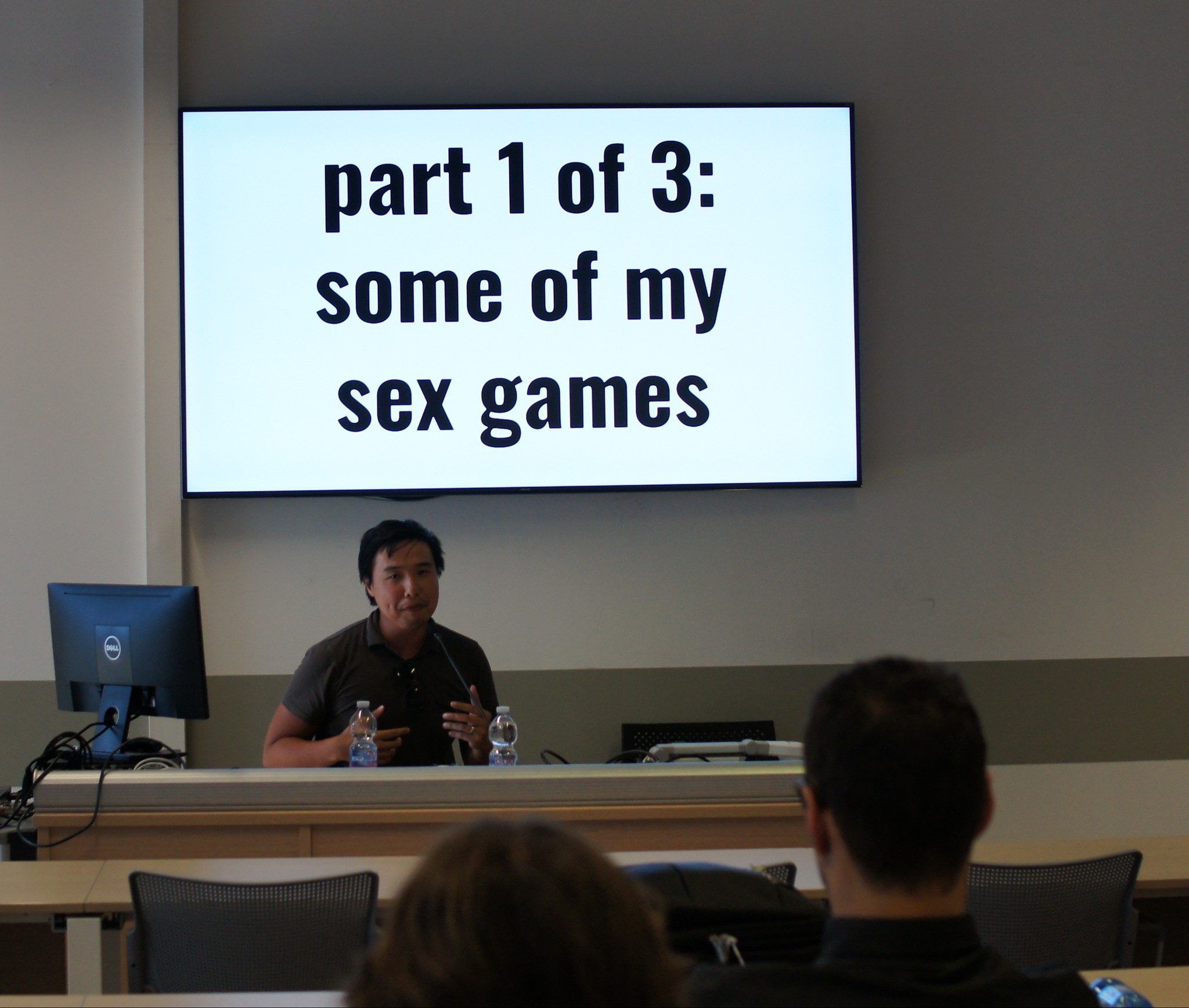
[45, 583, 209, 769]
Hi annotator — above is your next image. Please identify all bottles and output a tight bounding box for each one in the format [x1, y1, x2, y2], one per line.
[489, 706, 519, 765]
[347, 701, 378, 768]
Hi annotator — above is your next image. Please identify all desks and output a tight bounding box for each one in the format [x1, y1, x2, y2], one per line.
[0, 752, 1188, 1008]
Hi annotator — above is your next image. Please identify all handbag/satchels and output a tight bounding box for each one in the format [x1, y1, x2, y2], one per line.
[625, 859, 823, 965]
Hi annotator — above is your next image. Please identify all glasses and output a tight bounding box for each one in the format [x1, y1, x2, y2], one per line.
[793, 773, 814, 812]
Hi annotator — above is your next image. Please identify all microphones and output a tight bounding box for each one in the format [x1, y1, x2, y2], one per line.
[425, 617, 490, 728]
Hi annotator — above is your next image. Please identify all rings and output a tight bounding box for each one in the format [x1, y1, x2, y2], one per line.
[470, 725, 474, 734]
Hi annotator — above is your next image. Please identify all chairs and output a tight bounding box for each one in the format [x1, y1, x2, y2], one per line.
[128, 868, 378, 994]
[970, 848, 1148, 957]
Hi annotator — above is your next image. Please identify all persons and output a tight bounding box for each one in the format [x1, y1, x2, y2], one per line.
[261, 519, 497, 767]
[337, 821, 691, 1008]
[691, 653, 1101, 1008]
